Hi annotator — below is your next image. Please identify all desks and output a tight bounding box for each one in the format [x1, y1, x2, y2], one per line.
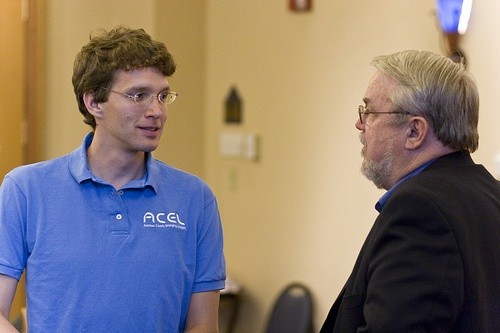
[219, 279, 247, 333]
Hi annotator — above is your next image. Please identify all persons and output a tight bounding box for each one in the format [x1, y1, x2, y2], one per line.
[0, 24, 226, 333]
[320, 49, 500, 333]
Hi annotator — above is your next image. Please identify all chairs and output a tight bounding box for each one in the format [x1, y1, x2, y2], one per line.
[260, 282, 317, 333]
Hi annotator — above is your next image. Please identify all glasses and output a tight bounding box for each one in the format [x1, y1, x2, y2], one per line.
[358, 104, 414, 123]
[98, 85, 177, 104]
